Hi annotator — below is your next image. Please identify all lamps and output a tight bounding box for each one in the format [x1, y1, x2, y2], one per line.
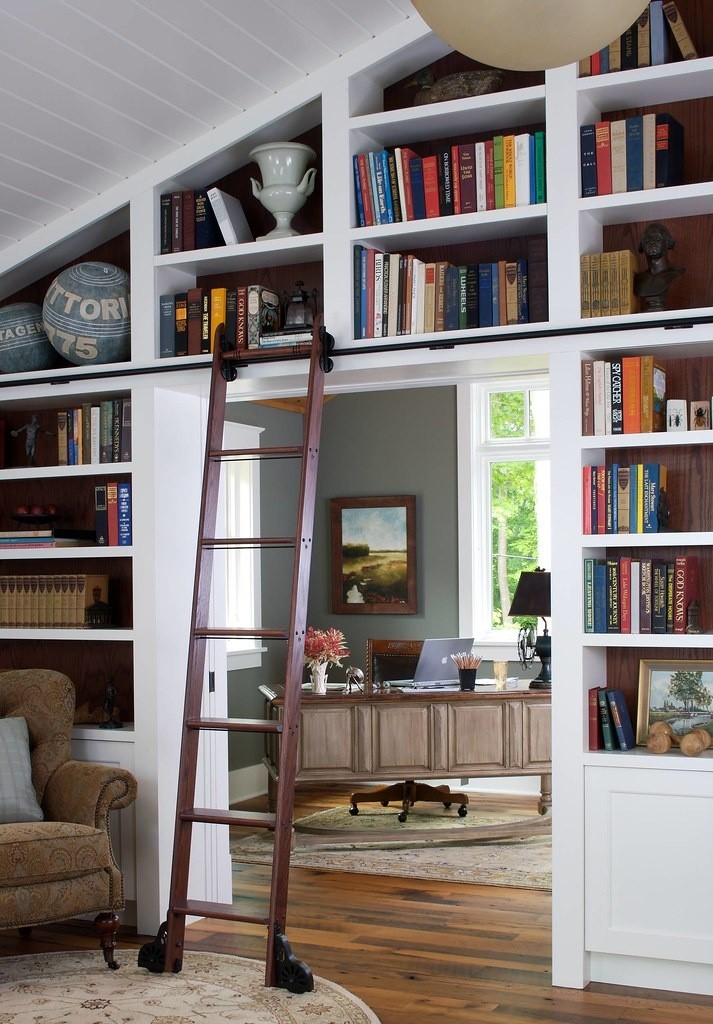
[508, 565, 553, 689]
[411, 0, 653, 72]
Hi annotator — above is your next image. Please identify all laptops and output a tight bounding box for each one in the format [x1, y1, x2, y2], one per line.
[388, 638, 475, 686]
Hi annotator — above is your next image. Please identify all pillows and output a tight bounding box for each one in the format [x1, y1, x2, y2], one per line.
[0, 716, 45, 824]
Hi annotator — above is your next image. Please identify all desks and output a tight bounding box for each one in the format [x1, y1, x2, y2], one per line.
[258, 678, 551, 831]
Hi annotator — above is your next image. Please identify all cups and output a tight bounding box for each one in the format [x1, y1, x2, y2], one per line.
[493, 659, 508, 687]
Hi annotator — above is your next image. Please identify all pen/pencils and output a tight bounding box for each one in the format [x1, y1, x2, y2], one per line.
[451, 651, 483, 668]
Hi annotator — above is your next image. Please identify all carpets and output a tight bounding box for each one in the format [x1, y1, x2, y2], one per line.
[226, 805, 553, 894]
[0, 948, 381, 1024]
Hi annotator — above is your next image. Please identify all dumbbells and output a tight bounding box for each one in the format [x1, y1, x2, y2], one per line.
[647, 720, 712, 757]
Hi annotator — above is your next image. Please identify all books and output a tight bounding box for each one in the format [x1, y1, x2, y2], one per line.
[589, 686, 636, 751]
[0, 574, 109, 629]
[353, 131, 545, 227]
[0, 528, 93, 549]
[160, 187, 254, 254]
[582, 355, 667, 436]
[579, 0, 698, 78]
[583, 463, 668, 535]
[94, 482, 132, 546]
[580, 249, 640, 319]
[57, 398, 131, 465]
[584, 556, 703, 634]
[580, 111, 685, 199]
[159, 284, 314, 358]
[354, 243, 528, 340]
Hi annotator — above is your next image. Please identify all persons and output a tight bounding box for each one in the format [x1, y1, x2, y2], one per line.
[11, 414, 57, 467]
[634, 224, 687, 295]
[102, 673, 118, 724]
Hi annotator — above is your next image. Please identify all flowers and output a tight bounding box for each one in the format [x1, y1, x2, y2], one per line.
[287, 627, 351, 669]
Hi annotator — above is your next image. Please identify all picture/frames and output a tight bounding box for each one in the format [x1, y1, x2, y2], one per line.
[634, 659, 713, 749]
[331, 495, 419, 616]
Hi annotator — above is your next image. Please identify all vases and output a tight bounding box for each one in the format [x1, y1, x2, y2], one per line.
[309, 661, 329, 697]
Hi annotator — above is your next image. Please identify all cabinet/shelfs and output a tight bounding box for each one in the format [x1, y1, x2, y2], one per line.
[552, 323, 713, 998]
[0, 375, 160, 945]
[0, 0, 713, 388]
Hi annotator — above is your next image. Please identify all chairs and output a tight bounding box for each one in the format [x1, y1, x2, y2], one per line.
[348, 637, 469, 824]
[0, 670, 137, 972]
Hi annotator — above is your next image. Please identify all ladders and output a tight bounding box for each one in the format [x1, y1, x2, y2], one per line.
[137, 323, 336, 996]
